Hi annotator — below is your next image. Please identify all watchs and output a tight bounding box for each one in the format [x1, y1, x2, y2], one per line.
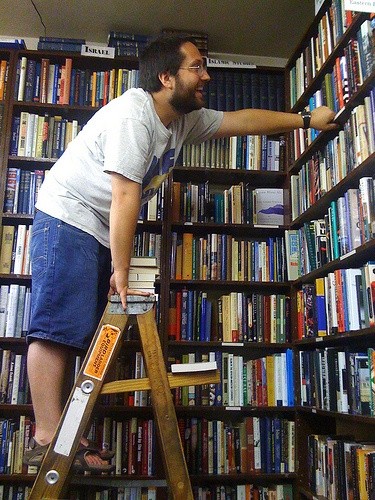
[302, 112, 313, 131]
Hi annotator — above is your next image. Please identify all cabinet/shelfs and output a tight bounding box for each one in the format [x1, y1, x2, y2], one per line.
[0, 0, 375, 500]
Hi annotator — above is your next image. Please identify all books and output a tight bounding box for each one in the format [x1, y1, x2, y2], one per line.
[10, 111, 84, 159]
[108, 256, 160, 298]
[0, 287, 288, 342]
[0, 224, 32, 280]
[0, 345, 297, 410]
[0, 475, 294, 500]
[288, 0, 375, 500]
[0, 417, 300, 473]
[138, 177, 288, 226]
[206, 67, 288, 112]
[4, 168, 49, 216]
[133, 231, 285, 283]
[184, 133, 285, 169]
[0, 57, 143, 107]
[38, 30, 207, 59]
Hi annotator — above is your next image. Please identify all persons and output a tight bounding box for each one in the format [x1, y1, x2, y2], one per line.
[25, 35, 334, 469]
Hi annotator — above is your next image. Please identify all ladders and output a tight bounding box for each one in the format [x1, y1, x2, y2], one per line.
[25, 294, 197, 499]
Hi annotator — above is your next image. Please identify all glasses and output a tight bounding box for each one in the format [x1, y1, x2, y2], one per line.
[178, 56, 208, 75]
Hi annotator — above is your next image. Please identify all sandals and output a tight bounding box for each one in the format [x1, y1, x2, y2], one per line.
[22, 437, 116, 473]
[80, 436, 115, 459]
